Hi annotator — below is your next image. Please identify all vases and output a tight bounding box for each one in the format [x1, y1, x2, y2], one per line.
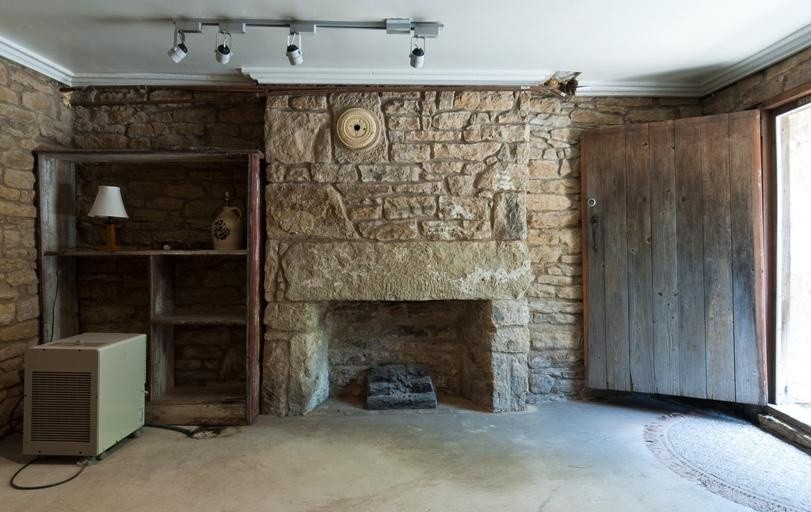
[211, 207, 245, 250]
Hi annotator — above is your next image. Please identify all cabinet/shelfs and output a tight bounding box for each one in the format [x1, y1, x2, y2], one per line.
[35, 148, 263, 424]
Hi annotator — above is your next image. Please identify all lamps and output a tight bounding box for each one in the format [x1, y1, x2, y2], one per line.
[167, 16, 445, 68]
[88, 186, 130, 250]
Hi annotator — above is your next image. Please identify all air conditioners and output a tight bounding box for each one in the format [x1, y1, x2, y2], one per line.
[23, 333, 147, 456]
